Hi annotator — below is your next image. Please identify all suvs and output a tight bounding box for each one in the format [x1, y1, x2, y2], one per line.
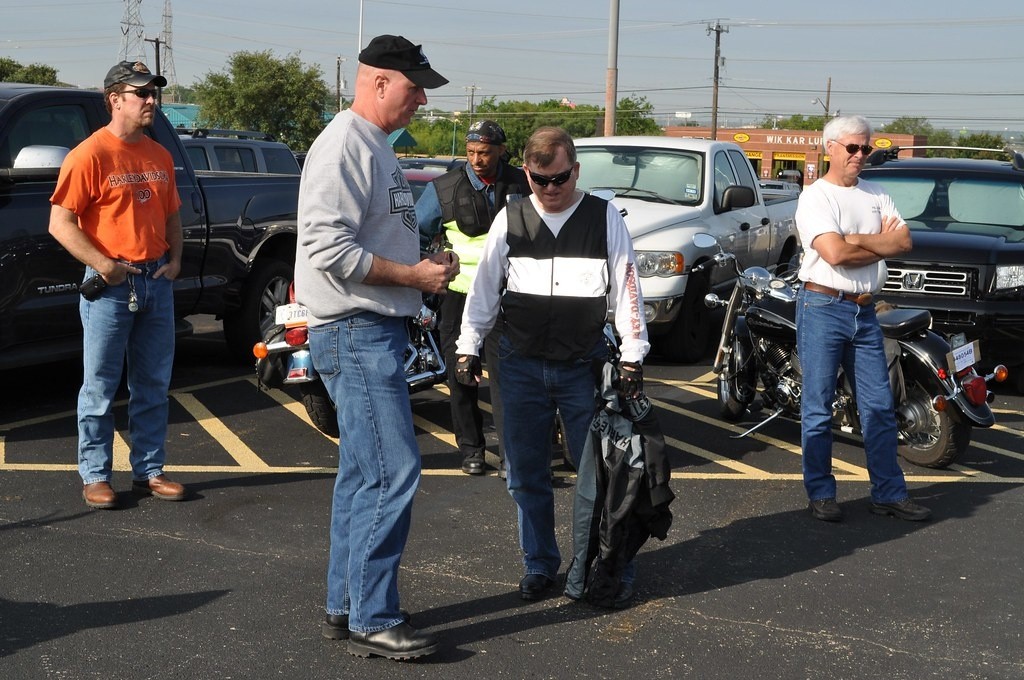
[855, 144, 1024, 395]
[177, 127, 303, 178]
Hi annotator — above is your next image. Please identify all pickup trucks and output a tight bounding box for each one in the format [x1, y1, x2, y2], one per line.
[563, 134, 802, 363]
[1, 83, 302, 380]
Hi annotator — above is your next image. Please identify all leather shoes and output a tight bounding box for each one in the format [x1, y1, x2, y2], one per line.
[132, 474, 188, 501]
[347, 622, 441, 660]
[83, 482, 120, 509]
[498, 460, 507, 479]
[611, 580, 635, 608]
[322, 607, 411, 639]
[518, 573, 550, 600]
[462, 452, 486, 473]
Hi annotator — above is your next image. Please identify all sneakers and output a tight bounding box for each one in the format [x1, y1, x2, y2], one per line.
[810, 498, 842, 521]
[871, 497, 933, 521]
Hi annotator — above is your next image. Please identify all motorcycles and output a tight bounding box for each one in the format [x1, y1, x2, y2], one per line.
[692, 232, 1009, 472]
[252, 242, 449, 438]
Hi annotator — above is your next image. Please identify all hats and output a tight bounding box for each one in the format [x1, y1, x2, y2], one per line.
[103, 60, 167, 88]
[358, 34, 449, 89]
[465, 120, 506, 144]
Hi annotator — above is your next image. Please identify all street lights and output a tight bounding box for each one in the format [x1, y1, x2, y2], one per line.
[811, 75, 834, 177]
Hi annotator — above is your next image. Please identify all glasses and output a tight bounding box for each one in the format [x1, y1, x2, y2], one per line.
[116, 88, 157, 99]
[527, 166, 574, 187]
[830, 140, 873, 155]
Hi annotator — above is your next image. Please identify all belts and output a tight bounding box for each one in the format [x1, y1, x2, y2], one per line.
[804, 282, 873, 306]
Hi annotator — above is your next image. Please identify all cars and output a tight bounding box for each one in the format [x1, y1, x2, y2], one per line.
[397, 153, 470, 203]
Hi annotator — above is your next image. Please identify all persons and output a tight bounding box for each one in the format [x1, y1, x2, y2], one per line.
[48, 60, 185, 508]
[414, 120, 533, 479]
[454, 126, 650, 600]
[292, 35, 461, 660]
[796, 116, 932, 520]
